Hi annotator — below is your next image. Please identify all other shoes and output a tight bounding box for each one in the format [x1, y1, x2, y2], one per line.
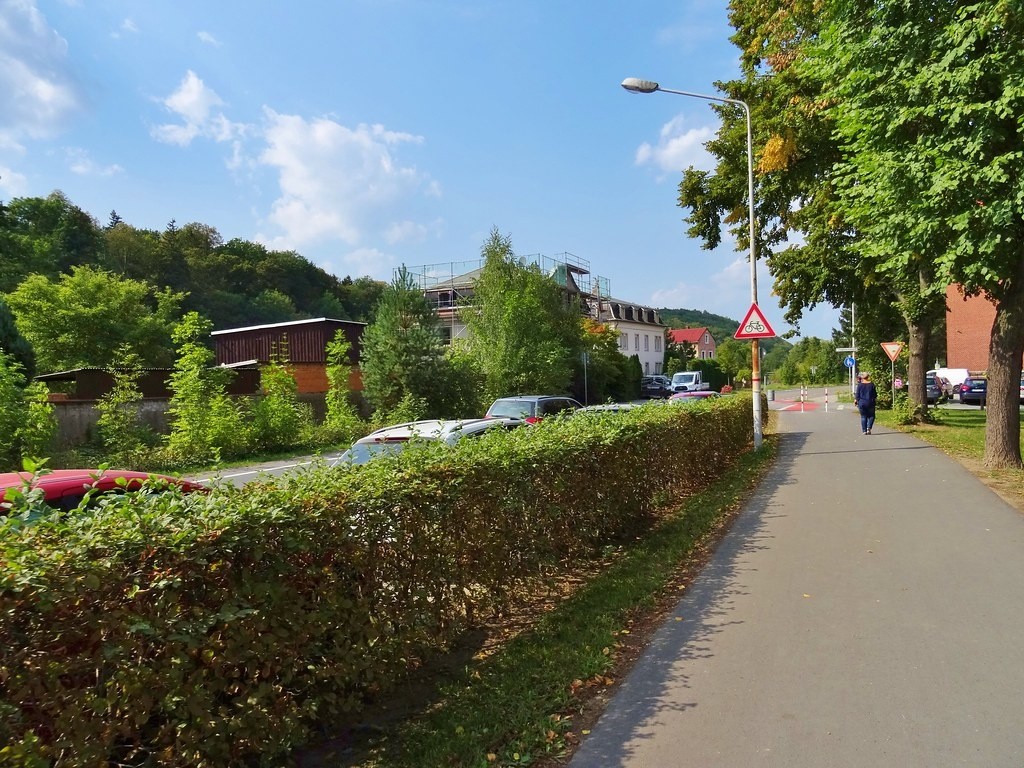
[867, 428, 871, 435]
[863, 432, 867, 435]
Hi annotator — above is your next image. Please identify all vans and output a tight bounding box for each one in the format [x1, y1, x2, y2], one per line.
[926, 368, 970, 394]
[1020, 371, 1024, 403]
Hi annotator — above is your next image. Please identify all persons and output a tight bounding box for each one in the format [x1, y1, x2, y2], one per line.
[854, 372, 878, 435]
[894, 375, 903, 388]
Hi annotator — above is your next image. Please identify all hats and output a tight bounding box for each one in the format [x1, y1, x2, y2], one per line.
[860, 372, 868, 378]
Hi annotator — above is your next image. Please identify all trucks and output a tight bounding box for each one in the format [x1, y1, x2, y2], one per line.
[666, 370, 709, 397]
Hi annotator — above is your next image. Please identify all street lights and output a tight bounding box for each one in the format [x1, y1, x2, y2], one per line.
[619, 77, 763, 454]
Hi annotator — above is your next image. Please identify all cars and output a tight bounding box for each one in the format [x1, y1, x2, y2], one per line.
[959, 376, 988, 405]
[939, 377, 955, 399]
[573, 403, 644, 416]
[667, 390, 722, 406]
[317, 412, 536, 476]
[926, 375, 941, 404]
[0, 469, 230, 523]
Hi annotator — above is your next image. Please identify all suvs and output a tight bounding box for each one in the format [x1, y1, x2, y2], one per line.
[482, 395, 585, 423]
